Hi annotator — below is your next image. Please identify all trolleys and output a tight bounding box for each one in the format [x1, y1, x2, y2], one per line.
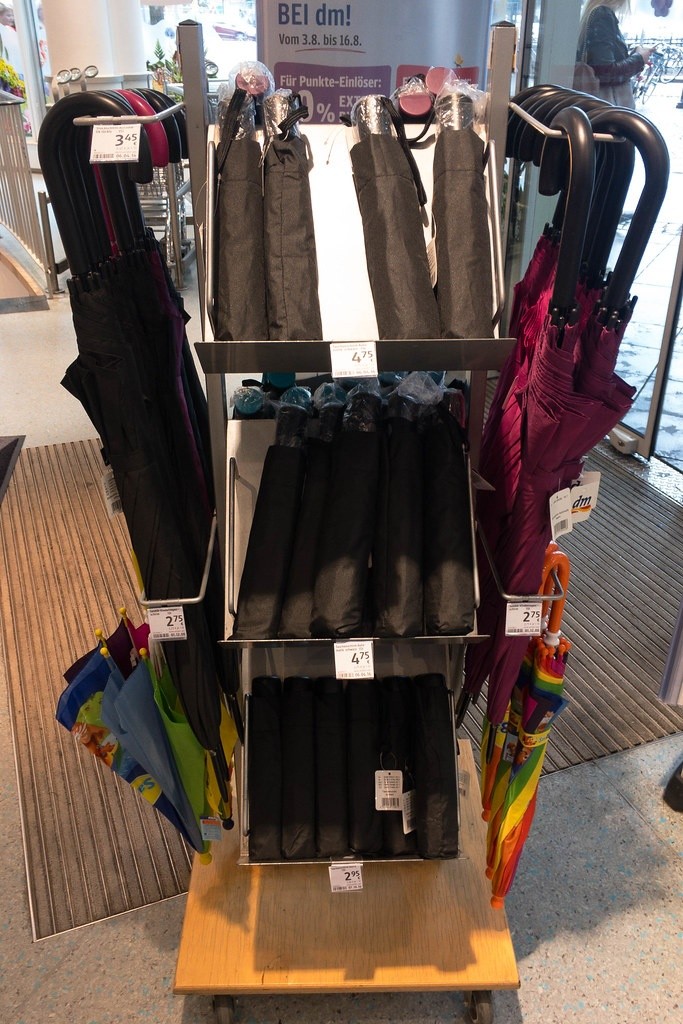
[132, 156, 198, 290]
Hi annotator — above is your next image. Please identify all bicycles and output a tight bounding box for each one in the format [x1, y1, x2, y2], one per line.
[625, 40, 683, 105]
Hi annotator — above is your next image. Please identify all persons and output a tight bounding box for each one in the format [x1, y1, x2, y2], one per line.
[573, 1, 657, 111]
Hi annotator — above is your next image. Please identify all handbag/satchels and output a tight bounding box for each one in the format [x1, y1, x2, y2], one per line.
[573, 61, 600, 98]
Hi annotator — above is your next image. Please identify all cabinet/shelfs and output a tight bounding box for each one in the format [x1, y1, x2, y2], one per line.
[78, 16, 636, 863]
